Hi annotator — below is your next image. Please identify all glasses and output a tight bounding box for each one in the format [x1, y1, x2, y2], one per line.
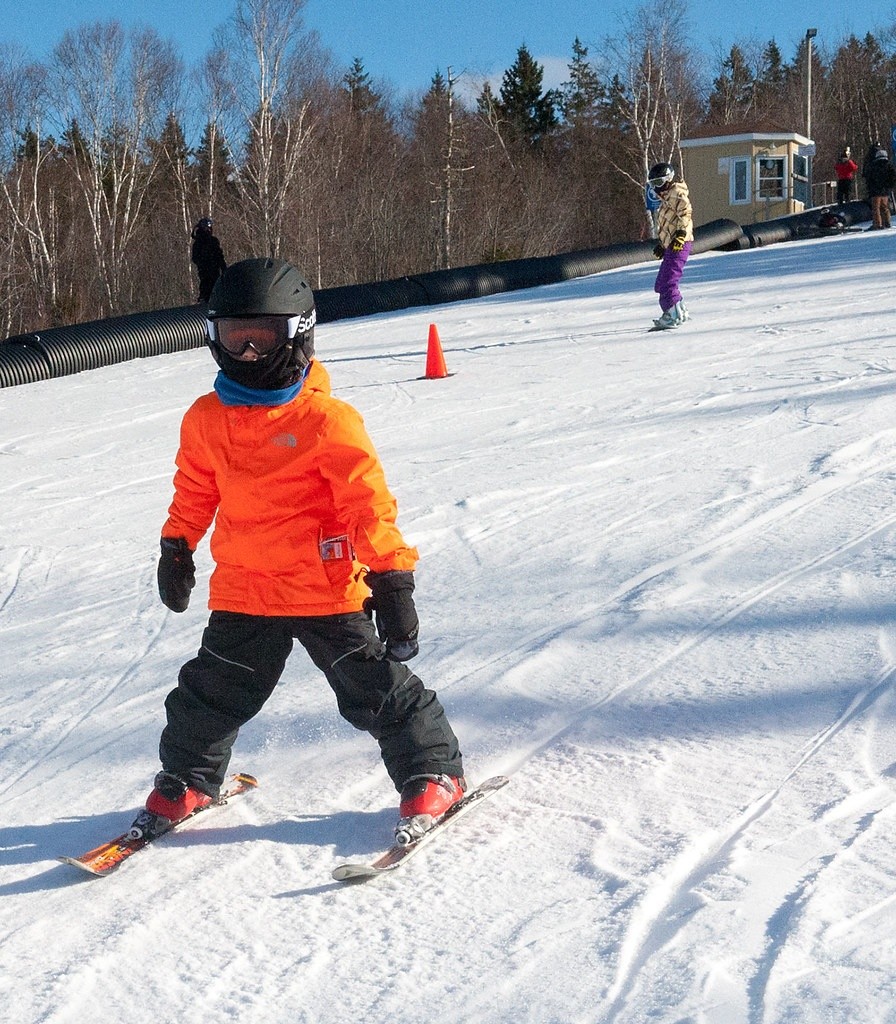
[203, 300, 316, 357]
[647, 169, 675, 189]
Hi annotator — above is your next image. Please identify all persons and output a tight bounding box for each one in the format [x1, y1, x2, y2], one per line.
[818, 207, 848, 230]
[649, 163, 694, 328]
[191, 218, 226, 304]
[834, 149, 859, 205]
[861, 141, 896, 230]
[128, 258, 467, 849]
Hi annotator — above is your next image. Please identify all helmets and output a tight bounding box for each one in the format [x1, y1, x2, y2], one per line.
[648, 163, 676, 193]
[205, 257, 316, 390]
[875, 150, 888, 160]
[198, 218, 213, 235]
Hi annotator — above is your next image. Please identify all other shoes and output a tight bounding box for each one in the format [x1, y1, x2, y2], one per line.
[883, 223, 891, 228]
[868, 225, 884, 231]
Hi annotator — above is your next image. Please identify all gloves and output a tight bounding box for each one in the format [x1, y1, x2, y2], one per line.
[669, 230, 686, 254]
[157, 536, 196, 613]
[653, 244, 666, 260]
[361, 570, 419, 661]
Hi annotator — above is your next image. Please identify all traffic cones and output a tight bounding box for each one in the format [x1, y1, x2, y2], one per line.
[426, 324, 448, 379]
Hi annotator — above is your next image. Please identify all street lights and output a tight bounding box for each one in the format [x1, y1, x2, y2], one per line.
[805, 29, 817, 208]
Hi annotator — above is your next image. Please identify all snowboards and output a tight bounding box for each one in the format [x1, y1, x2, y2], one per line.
[651, 312, 691, 331]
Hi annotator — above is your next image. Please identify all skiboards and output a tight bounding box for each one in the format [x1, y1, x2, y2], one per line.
[56, 769, 257, 879]
[331, 774, 510, 881]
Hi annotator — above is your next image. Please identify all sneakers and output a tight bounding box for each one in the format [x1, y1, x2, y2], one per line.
[394, 774, 467, 847]
[653, 300, 689, 329]
[146, 770, 214, 823]
